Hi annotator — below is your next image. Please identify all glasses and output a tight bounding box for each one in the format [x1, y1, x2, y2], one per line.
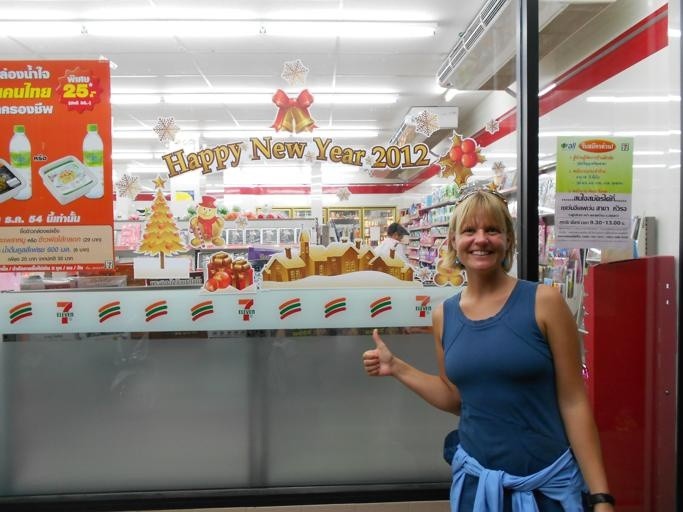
[458, 189, 507, 207]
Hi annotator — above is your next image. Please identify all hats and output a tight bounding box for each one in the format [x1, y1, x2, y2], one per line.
[389, 223, 409, 235]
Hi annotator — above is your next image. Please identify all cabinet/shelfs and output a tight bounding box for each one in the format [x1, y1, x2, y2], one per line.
[397, 187, 516, 269]
[256, 207, 398, 250]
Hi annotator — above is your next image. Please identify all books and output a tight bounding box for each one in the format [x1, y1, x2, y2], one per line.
[542, 255, 580, 300]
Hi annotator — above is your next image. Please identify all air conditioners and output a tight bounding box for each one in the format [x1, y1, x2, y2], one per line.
[369, 106, 459, 180]
[435, 0, 615, 98]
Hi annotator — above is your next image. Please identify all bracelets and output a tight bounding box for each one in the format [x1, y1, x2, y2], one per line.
[587, 492, 616, 507]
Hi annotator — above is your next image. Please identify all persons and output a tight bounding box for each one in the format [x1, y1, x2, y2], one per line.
[361, 190, 618, 511]
[373, 221, 409, 263]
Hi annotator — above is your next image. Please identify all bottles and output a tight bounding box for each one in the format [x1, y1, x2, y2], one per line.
[80, 123, 104, 200]
[7, 124, 32, 201]
